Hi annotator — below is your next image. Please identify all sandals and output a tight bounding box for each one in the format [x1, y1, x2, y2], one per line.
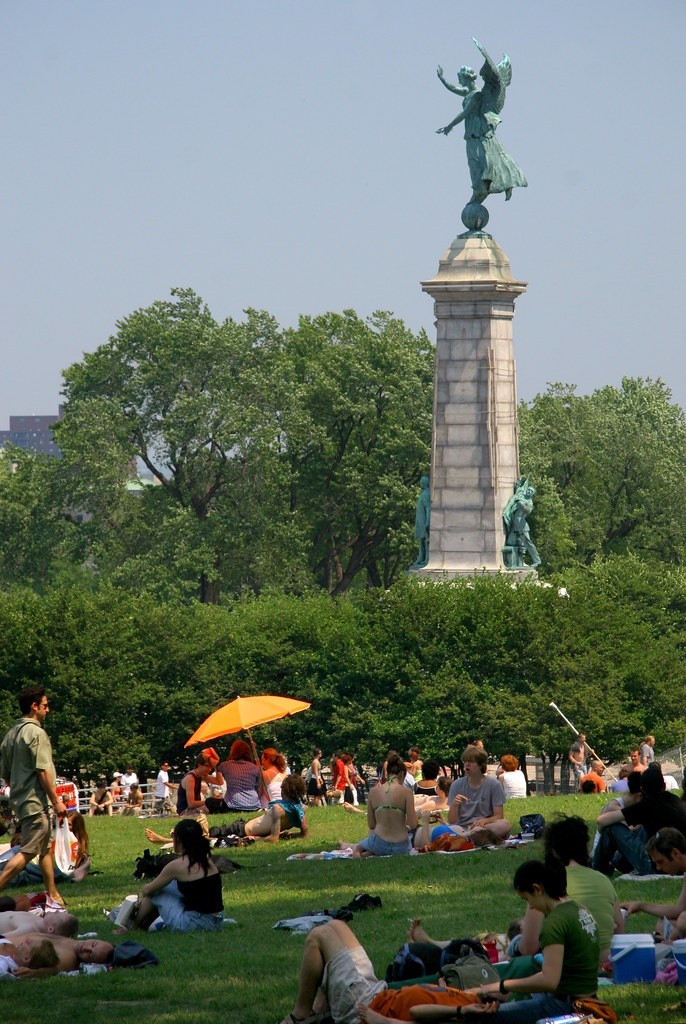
[279, 1013, 317, 1024]
[314, 1009, 332, 1021]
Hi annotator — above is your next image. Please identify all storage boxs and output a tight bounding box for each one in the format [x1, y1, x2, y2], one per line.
[609, 933, 656, 984]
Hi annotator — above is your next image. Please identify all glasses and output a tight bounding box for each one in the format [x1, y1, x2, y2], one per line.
[38, 704, 48, 710]
[655, 831, 666, 841]
[318, 752, 323, 754]
[200, 763, 212, 769]
[130, 787, 134, 790]
[163, 765, 169, 767]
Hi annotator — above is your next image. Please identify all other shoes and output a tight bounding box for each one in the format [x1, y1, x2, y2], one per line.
[340, 893, 382, 913]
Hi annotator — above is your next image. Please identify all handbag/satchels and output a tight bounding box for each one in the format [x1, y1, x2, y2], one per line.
[438, 938, 492, 968]
[573, 998, 617, 1024]
[437, 945, 501, 992]
[386, 940, 442, 983]
[55, 814, 79, 876]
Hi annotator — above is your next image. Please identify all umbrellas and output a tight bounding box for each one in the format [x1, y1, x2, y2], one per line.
[184, 696, 311, 801]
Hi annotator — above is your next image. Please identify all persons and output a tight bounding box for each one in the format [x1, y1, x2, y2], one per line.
[0, 685, 71, 910]
[590, 761, 686, 877]
[504, 475, 542, 567]
[111, 818, 226, 935]
[279, 814, 626, 1024]
[436, 39, 528, 206]
[0, 733, 531, 884]
[619, 828, 686, 943]
[570, 733, 679, 794]
[413, 476, 430, 566]
[0, 894, 119, 985]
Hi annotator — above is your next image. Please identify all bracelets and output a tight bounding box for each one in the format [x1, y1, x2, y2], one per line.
[500, 979, 509, 995]
[457, 1004, 463, 1017]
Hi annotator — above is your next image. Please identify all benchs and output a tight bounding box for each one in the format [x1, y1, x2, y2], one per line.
[76, 783, 163, 816]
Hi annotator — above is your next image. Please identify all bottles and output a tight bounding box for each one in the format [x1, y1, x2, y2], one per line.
[79, 962, 115, 976]
[537, 1012, 585, 1024]
[312, 853, 335, 860]
[223, 918, 237, 924]
[212, 785, 223, 798]
[76, 932, 99, 939]
[56, 775, 66, 785]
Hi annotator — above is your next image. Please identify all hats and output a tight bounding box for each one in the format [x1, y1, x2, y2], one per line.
[113, 772, 123, 779]
